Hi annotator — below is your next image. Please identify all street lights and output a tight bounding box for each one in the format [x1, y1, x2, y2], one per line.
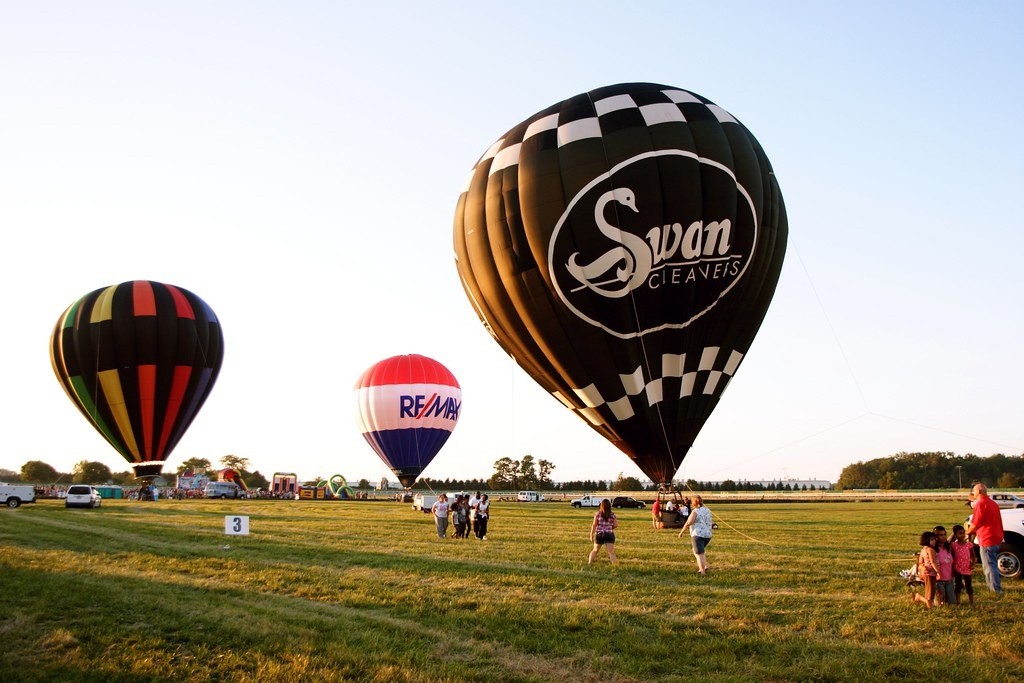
[956, 466, 963, 488]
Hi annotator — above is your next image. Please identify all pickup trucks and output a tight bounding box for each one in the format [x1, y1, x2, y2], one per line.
[570, 495, 612, 509]
[0, 482, 35, 507]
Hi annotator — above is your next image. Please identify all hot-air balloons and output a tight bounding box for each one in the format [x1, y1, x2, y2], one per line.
[452, 80, 788, 490]
[48, 278, 225, 478]
[351, 353, 462, 490]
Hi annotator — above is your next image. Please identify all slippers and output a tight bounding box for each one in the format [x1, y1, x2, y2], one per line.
[910, 592, 915, 602]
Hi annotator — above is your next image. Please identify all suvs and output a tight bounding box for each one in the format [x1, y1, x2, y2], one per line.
[66, 485, 103, 508]
[612, 497, 645, 509]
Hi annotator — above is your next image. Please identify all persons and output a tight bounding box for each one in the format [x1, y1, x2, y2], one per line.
[36, 484, 69, 497]
[432, 491, 490, 540]
[122, 486, 205, 501]
[395, 492, 412, 503]
[651, 497, 679, 529]
[679, 495, 712, 575]
[915, 483, 1004, 609]
[588, 499, 618, 565]
[234, 486, 299, 500]
[354, 491, 369, 498]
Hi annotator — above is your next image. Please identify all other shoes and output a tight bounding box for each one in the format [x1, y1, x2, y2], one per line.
[474, 535, 478, 540]
[483, 536, 488, 540]
[698, 566, 708, 573]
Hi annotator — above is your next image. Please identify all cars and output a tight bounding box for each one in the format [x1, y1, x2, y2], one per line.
[965, 509, 1024, 578]
[205, 483, 250, 499]
[518, 491, 545, 502]
[970, 493, 1024, 509]
